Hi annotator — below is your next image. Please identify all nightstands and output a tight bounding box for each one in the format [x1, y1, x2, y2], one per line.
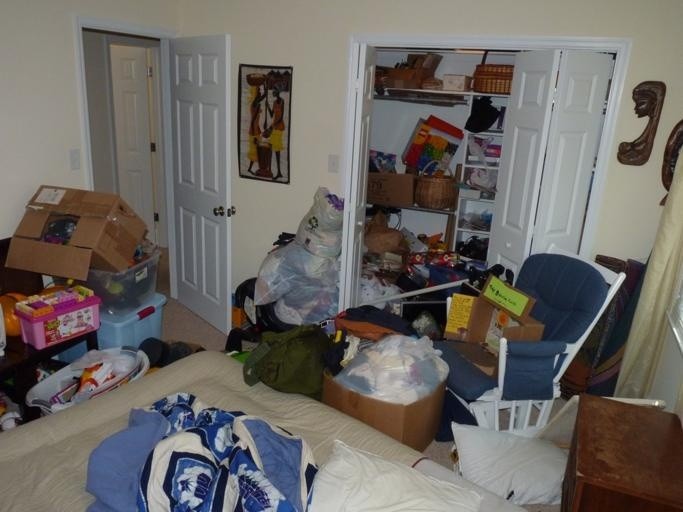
[564, 392, 681, 510]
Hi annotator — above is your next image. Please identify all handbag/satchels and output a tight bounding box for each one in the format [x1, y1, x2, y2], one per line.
[365, 209, 402, 254]
[253, 186, 344, 326]
[254, 324, 334, 395]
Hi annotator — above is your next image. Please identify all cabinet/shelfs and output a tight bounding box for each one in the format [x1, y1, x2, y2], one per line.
[338, 38, 630, 316]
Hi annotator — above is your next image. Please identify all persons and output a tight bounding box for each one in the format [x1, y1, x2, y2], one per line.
[262, 80, 285, 180]
[246, 80, 269, 175]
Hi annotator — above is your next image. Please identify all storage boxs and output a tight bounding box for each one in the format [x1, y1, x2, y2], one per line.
[316, 351, 450, 453]
[441, 64, 514, 94]
[366, 169, 418, 209]
[444, 276, 544, 379]
[3, 186, 164, 415]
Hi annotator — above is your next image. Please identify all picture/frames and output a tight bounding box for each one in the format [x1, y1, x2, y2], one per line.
[239, 63, 293, 184]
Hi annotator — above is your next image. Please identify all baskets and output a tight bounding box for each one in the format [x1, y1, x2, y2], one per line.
[415, 159, 457, 209]
[473, 64, 514, 95]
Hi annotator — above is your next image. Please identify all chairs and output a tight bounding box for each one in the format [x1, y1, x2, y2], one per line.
[442, 240, 627, 439]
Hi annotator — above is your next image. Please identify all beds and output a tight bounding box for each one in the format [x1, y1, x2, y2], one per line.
[0, 348, 537, 512]
